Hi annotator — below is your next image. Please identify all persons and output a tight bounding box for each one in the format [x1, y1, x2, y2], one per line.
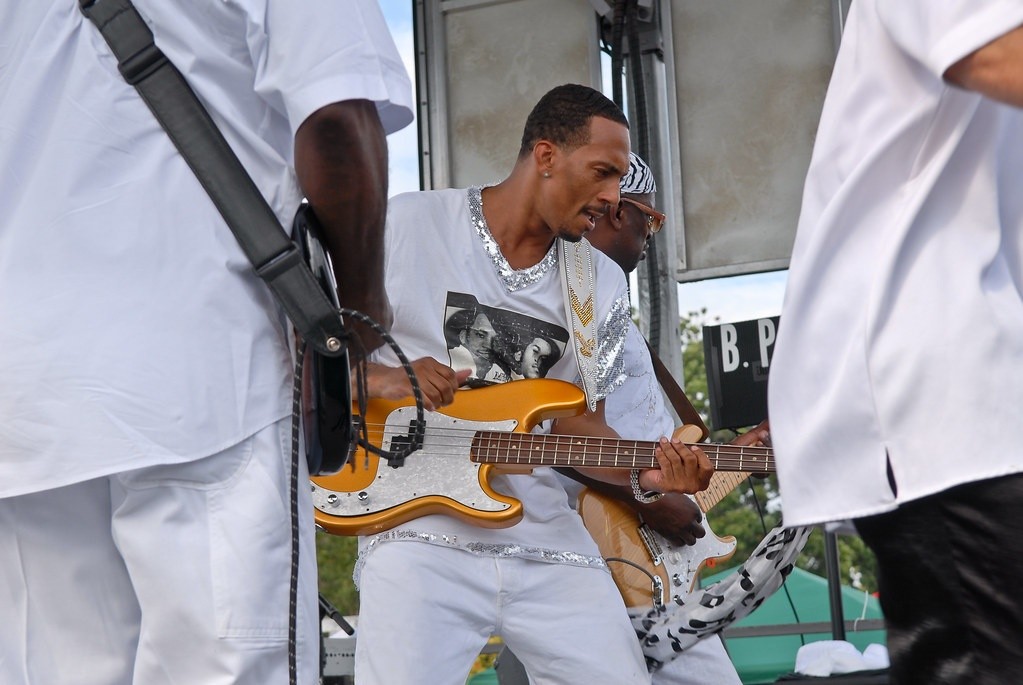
[587, 0, 1023, 685]
[350, 83, 713, 685]
[0, 0, 414, 685]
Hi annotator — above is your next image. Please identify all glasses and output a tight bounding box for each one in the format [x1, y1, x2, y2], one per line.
[620, 197, 666, 233]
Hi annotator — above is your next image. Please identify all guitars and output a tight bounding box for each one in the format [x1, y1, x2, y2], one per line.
[575, 423, 754, 609]
[308, 378, 778, 538]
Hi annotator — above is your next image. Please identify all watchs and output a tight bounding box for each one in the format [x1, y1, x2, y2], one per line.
[626, 467, 664, 503]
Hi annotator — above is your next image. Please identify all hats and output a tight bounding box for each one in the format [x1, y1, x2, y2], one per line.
[619, 151, 657, 194]
